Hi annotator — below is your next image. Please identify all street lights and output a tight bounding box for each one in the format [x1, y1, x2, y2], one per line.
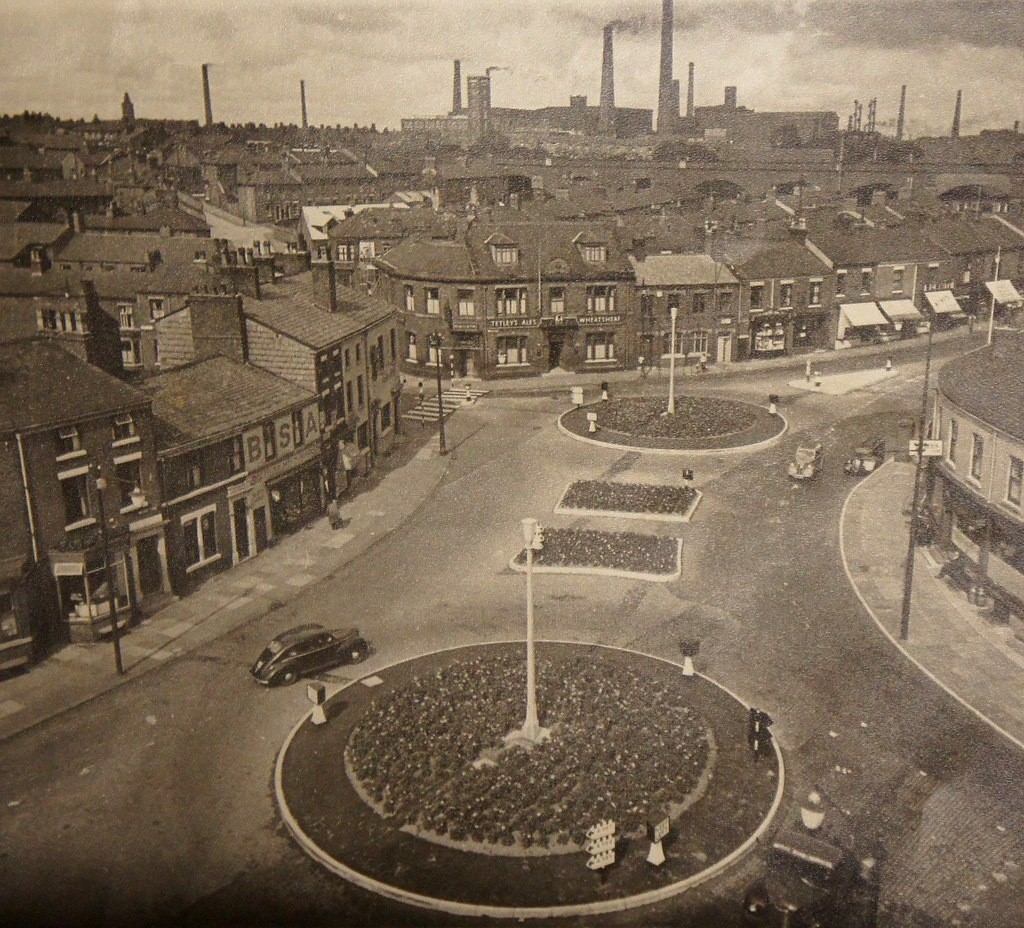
[856, 448, 923, 640]
[89, 456, 148, 678]
[801, 782, 885, 928]
[431, 329, 508, 456]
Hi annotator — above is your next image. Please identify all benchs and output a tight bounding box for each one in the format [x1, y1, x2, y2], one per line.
[950, 571, 977, 593]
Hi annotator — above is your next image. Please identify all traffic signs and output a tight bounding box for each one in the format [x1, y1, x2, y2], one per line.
[585, 822, 615, 871]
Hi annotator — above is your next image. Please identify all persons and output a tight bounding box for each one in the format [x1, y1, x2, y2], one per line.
[936, 552, 962, 579]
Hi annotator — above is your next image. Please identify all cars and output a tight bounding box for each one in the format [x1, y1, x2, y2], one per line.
[844, 454, 883, 476]
[788, 439, 824, 484]
[249, 623, 369, 688]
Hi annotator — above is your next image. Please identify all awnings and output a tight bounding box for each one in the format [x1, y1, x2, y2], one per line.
[926, 290, 962, 313]
[839, 303, 889, 327]
[879, 298, 923, 321]
[984, 280, 1024, 305]
